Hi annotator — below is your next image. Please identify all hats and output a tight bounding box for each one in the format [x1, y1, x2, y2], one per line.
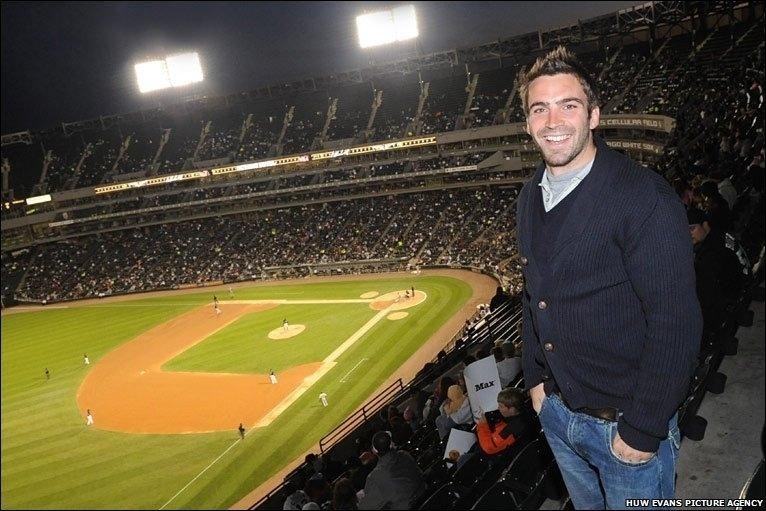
[372, 431, 393, 455]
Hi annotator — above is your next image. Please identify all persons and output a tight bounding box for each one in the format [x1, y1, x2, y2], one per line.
[83, 353, 90, 366]
[282, 318, 288, 332]
[86, 409, 93, 426]
[44, 368, 50, 379]
[238, 423, 246, 440]
[516, 44, 708, 511]
[268, 370, 277, 385]
[214, 304, 221, 315]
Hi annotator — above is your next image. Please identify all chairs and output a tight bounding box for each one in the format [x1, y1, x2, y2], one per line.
[348, 270, 766, 511]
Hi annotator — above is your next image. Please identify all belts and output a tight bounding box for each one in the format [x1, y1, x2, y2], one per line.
[551, 387, 619, 422]
